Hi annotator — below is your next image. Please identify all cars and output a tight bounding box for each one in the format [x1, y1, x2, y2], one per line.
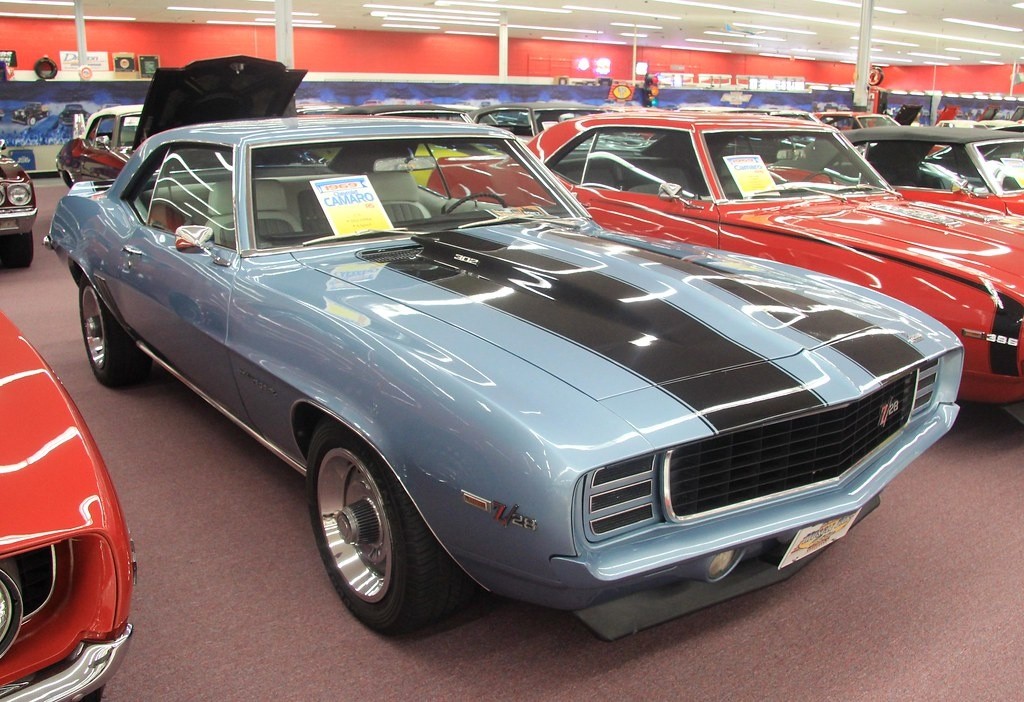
[41, 56, 963, 644]
[778, 98, 1024, 226]
[55, 56, 308, 187]
[434, 118, 1024, 413]
[474, 103, 604, 147]
[0, 312, 142, 702]
[295, 102, 508, 182]
[0, 139, 38, 272]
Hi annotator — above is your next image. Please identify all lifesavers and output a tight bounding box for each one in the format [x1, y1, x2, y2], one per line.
[34, 57, 58, 79]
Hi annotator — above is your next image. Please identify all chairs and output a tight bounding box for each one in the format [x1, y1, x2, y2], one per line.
[611, 159, 740, 198]
[362, 171, 431, 222]
[206, 180, 304, 250]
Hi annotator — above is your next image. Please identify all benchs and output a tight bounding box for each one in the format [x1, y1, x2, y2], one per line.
[144, 174, 359, 234]
[554, 157, 696, 196]
